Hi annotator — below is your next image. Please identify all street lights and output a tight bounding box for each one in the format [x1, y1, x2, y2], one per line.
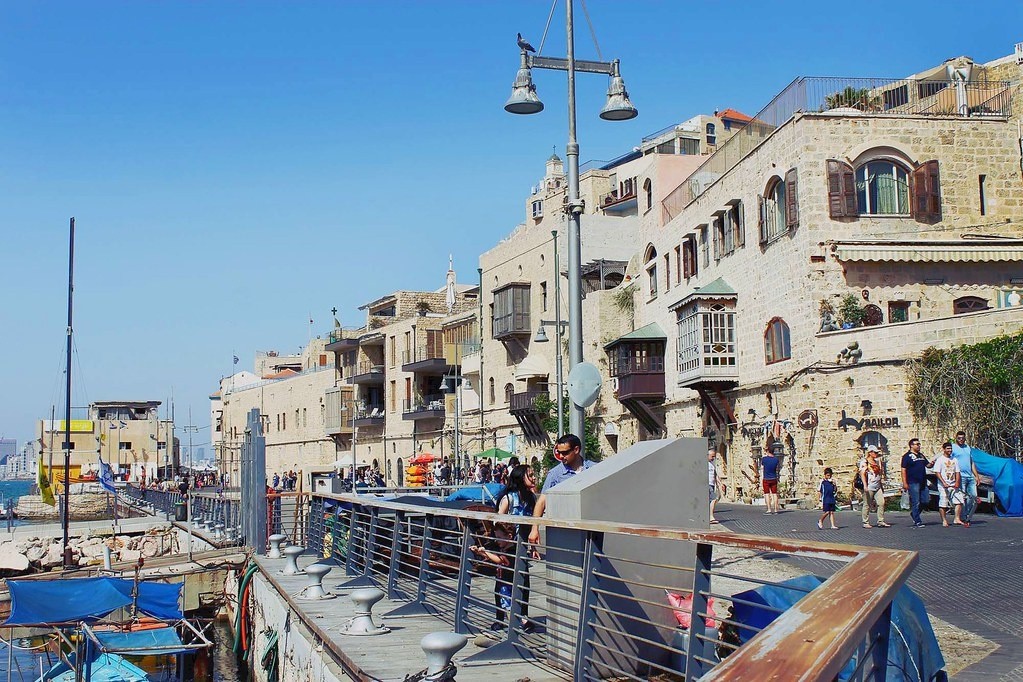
[339, 373, 368, 491]
[182, 404, 200, 478]
[438, 333, 474, 492]
[503, 0, 639, 462]
[533, 253, 570, 439]
[260, 387, 271, 437]
[170, 401, 177, 482]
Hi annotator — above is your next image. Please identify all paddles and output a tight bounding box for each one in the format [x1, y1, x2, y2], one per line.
[48, 638, 85, 680]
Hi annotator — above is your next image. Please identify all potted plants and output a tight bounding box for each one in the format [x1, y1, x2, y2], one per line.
[415, 302, 433, 317]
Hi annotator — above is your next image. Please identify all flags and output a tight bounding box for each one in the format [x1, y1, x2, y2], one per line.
[233, 356, 239, 364]
[99, 460, 116, 494]
[38, 457, 55, 506]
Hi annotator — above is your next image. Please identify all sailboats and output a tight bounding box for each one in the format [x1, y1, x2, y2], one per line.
[0, 215, 214, 682]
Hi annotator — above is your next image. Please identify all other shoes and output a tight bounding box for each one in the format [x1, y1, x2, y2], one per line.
[763, 511, 772, 515]
[710, 520, 719, 524]
[522, 620, 536, 634]
[862, 523, 872, 528]
[917, 521, 925, 528]
[878, 521, 890, 527]
[774, 512, 778, 515]
[491, 622, 508, 631]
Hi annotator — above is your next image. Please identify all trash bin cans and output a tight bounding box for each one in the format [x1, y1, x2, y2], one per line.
[543, 435, 710, 678]
[174, 503, 187, 521]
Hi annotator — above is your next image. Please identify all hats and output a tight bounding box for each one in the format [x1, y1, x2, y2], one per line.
[765, 446, 774, 451]
[867, 445, 881, 452]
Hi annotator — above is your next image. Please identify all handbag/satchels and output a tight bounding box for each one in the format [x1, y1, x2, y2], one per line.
[854, 458, 868, 489]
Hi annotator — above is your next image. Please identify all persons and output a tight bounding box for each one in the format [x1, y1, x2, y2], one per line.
[470, 465, 537, 632]
[817, 468, 839, 530]
[933, 442, 965, 527]
[950, 432, 980, 525]
[901, 439, 935, 527]
[528, 434, 600, 544]
[178, 478, 191, 498]
[348, 466, 386, 493]
[707, 450, 720, 524]
[761, 446, 779, 516]
[273, 470, 297, 492]
[175, 472, 230, 488]
[139, 467, 168, 493]
[431, 462, 465, 496]
[859, 445, 891, 528]
[468, 460, 520, 483]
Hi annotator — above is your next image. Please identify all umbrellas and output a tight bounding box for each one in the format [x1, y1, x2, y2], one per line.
[408, 453, 441, 463]
[331, 453, 368, 469]
[475, 447, 515, 459]
[447, 270, 456, 312]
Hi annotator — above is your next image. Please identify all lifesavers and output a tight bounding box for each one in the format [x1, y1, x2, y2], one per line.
[139, 537, 158, 557]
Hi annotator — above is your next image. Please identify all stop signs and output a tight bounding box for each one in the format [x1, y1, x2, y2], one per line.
[553, 443, 563, 461]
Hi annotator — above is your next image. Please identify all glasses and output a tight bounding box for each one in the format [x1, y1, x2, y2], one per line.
[557, 448, 572, 455]
[958, 437, 965, 440]
[911, 443, 921, 446]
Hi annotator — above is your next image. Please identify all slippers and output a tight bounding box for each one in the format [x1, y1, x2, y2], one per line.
[952, 522, 964, 526]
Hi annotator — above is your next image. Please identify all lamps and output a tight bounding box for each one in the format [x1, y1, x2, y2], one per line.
[697, 412, 702, 417]
[748, 408, 755, 414]
